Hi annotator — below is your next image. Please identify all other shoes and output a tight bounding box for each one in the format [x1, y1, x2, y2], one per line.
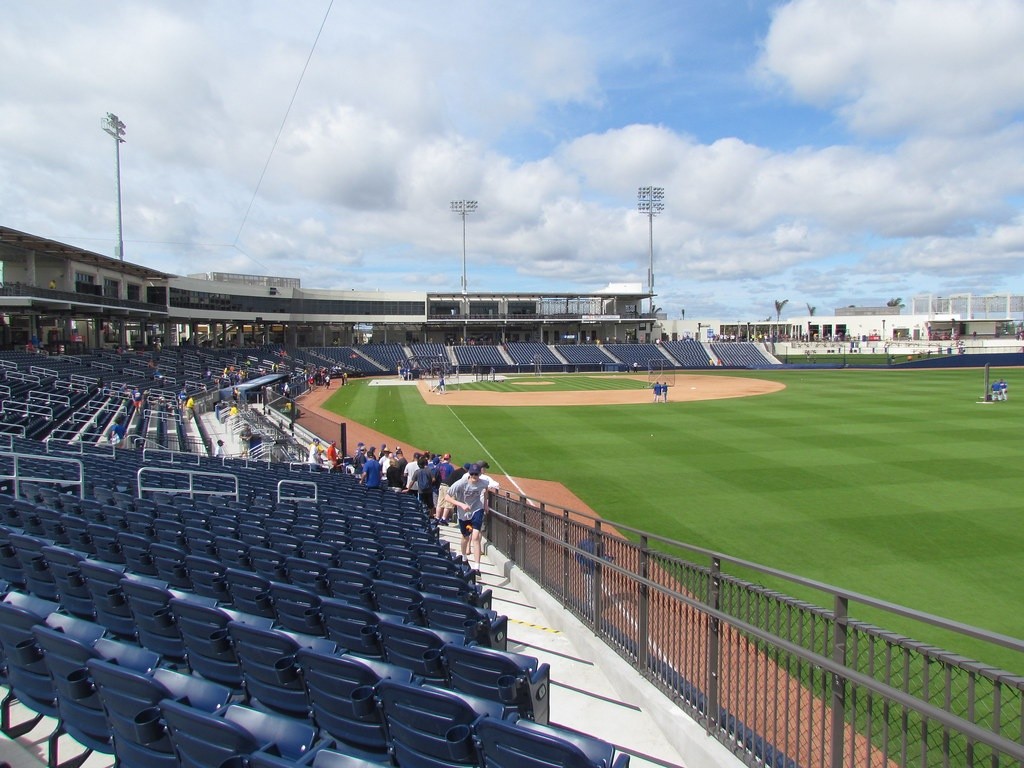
[466, 550, 471, 555]
[439, 519, 448, 526]
[480, 552, 485, 555]
[475, 570, 482, 580]
[433, 518, 439, 524]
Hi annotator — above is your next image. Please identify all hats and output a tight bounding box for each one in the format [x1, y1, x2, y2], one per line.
[479, 461, 489, 468]
[217, 440, 224, 444]
[357, 442, 365, 446]
[367, 451, 373, 458]
[396, 451, 402, 455]
[433, 457, 440, 464]
[469, 463, 480, 473]
[444, 454, 451, 460]
[381, 447, 392, 453]
[463, 463, 470, 469]
[313, 438, 321, 442]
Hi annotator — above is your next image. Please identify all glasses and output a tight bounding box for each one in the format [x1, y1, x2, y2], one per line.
[470, 473, 478, 476]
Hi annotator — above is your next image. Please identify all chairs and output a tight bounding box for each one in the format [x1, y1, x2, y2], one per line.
[0, 344, 772, 768]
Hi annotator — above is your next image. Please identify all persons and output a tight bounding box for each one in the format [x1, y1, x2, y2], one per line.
[153, 338, 162, 351]
[653, 381, 669, 404]
[437, 377, 446, 396]
[49, 279, 56, 289]
[256, 362, 348, 419]
[111, 420, 128, 449]
[179, 365, 249, 423]
[26, 334, 43, 353]
[309, 436, 499, 580]
[216, 439, 225, 457]
[633, 361, 637, 373]
[576, 532, 612, 612]
[398, 364, 405, 381]
[991, 378, 1009, 402]
[354, 333, 664, 346]
[279, 351, 287, 359]
[239, 424, 253, 457]
[678, 327, 1024, 366]
[128, 387, 146, 414]
[147, 360, 164, 381]
[115, 346, 122, 356]
[155, 396, 176, 418]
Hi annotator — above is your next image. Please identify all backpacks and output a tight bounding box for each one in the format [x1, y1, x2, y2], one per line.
[416, 469, 432, 491]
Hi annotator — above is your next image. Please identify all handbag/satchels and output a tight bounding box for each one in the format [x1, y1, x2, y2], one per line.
[111, 433, 119, 445]
